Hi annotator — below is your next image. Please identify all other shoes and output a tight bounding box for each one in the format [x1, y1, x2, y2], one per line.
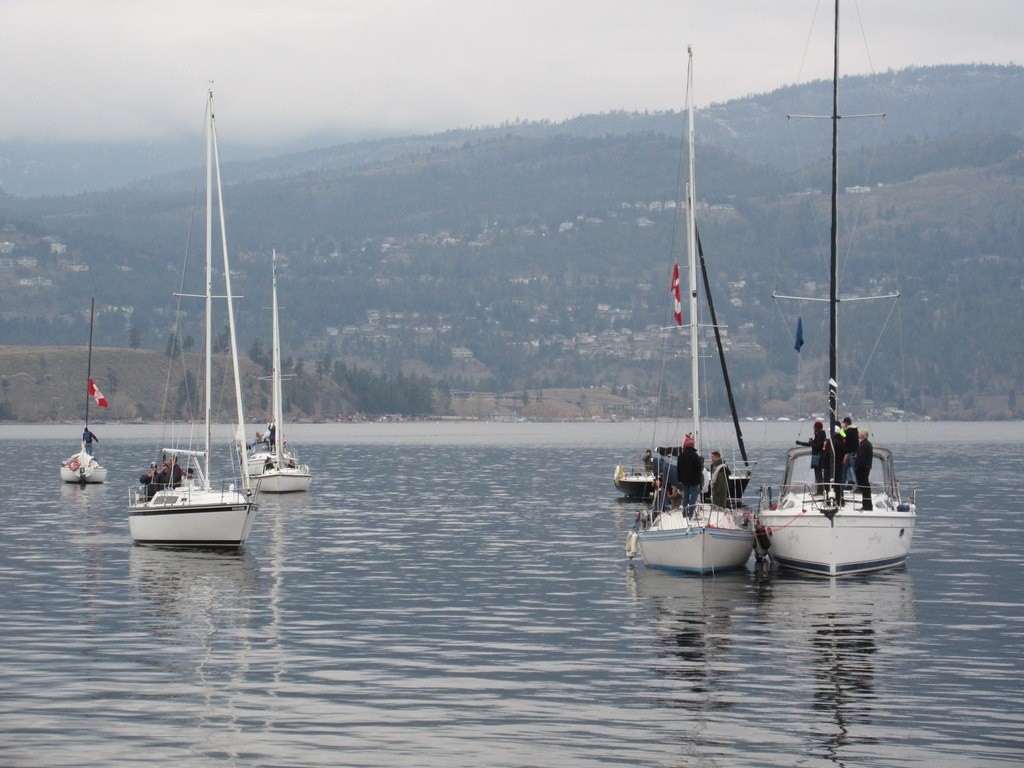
[843, 485, 852, 490]
[850, 488, 862, 493]
[808, 491, 823, 496]
[856, 508, 872, 511]
[826, 497, 845, 507]
[683, 512, 693, 518]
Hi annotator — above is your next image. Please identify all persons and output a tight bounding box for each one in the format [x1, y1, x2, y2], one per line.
[795, 422, 826, 495]
[83, 428, 100, 455]
[825, 417, 861, 506]
[855, 431, 873, 511]
[140, 455, 194, 501]
[710, 452, 731, 508]
[676, 438, 701, 517]
[265, 457, 273, 470]
[268, 425, 275, 451]
[642, 450, 654, 476]
[652, 473, 669, 525]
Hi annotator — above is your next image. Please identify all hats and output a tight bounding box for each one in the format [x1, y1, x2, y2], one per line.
[828, 425, 841, 432]
[815, 422, 823, 428]
[685, 432, 692, 438]
[150, 462, 159, 468]
[163, 462, 170, 468]
[842, 417, 851, 423]
[683, 438, 696, 448]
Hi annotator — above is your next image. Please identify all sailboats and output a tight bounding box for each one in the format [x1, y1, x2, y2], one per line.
[128, 93, 261, 553]
[59, 298, 107, 484]
[755, 1, 918, 580]
[243, 245, 313, 495]
[615, 47, 757, 579]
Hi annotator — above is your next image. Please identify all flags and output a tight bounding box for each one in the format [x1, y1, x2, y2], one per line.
[89, 379, 107, 408]
[794, 318, 804, 353]
[671, 263, 681, 326]
